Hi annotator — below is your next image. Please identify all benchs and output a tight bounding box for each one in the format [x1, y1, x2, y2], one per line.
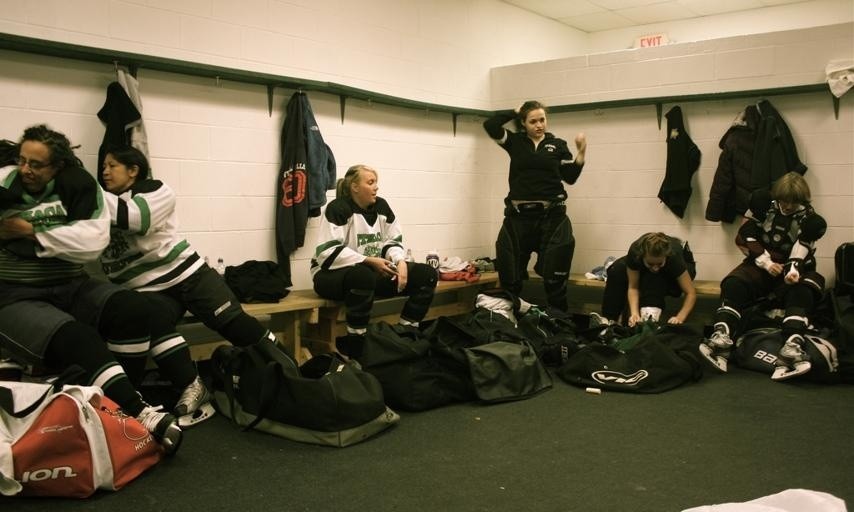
[520, 268, 726, 325]
[300, 270, 503, 370]
[176, 293, 321, 377]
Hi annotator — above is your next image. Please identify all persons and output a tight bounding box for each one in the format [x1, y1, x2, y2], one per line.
[482, 99, 587, 311]
[600, 231, 699, 328]
[695, 169, 828, 381]
[96, 143, 299, 418]
[310, 164, 440, 337]
[0, 122, 183, 461]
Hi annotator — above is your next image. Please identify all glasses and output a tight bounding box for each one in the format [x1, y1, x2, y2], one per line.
[13, 155, 58, 169]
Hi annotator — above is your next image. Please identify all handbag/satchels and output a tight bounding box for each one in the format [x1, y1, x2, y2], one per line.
[203, 311, 552, 447]
[0, 377, 162, 501]
[518, 307, 710, 394]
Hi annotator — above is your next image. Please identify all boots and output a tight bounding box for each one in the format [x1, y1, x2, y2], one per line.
[695, 320, 738, 374]
[172, 371, 215, 428]
[133, 401, 184, 458]
[769, 333, 812, 381]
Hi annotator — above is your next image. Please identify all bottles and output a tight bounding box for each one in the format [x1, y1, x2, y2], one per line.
[403, 249, 414, 262]
[425, 250, 439, 283]
